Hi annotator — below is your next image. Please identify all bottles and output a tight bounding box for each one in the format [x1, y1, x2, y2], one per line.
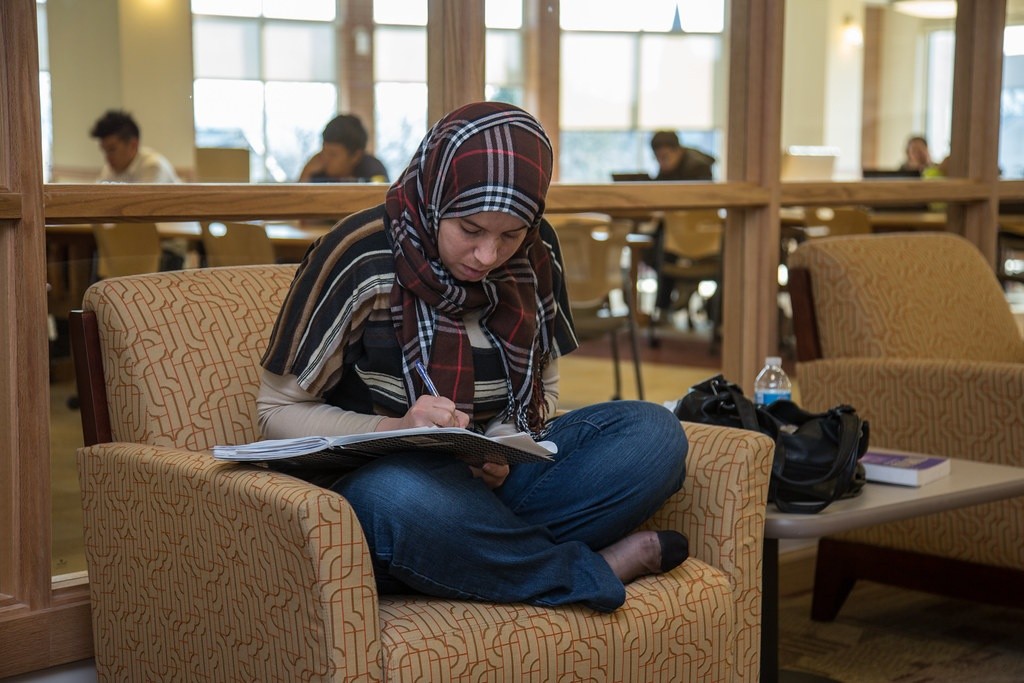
[754, 356, 792, 410]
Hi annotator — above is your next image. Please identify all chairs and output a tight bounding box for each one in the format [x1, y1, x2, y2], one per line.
[52, 207, 875, 402]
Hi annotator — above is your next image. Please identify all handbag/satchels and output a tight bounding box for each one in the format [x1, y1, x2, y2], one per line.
[674, 373, 869, 514]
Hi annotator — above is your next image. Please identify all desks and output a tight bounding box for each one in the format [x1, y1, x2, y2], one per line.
[778, 205, 949, 227]
[544, 208, 656, 331]
[157, 218, 340, 261]
[761, 446, 1024, 683]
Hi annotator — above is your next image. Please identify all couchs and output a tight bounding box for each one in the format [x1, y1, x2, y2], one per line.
[786, 231, 1024, 624]
[68, 262, 775, 683]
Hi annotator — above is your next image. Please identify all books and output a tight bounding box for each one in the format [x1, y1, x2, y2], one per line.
[213, 426, 555, 480]
[859, 452, 951, 487]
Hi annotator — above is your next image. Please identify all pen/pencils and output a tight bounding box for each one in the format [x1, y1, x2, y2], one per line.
[414, 359, 441, 398]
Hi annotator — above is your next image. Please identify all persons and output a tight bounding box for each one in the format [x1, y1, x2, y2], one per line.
[901, 137, 950, 210]
[299, 114, 390, 226]
[256, 101, 689, 612]
[88, 109, 187, 271]
[642, 131, 716, 324]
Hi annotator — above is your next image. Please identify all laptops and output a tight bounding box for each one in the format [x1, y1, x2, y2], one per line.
[612, 172, 651, 181]
[861, 170, 931, 212]
